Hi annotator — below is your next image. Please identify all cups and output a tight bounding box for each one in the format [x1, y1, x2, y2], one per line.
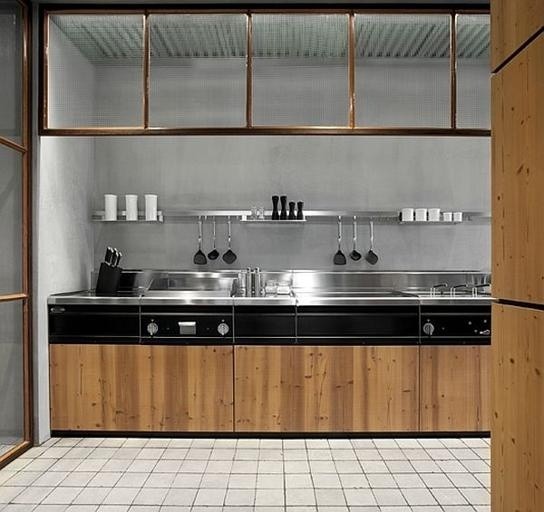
[443, 212, 452, 221]
[416, 209, 427, 221]
[428, 208, 441, 222]
[402, 208, 414, 221]
[453, 213, 463, 220]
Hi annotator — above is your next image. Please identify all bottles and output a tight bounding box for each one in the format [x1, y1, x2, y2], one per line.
[145, 194, 158, 221]
[104, 193, 117, 220]
[125, 195, 138, 220]
[237, 266, 265, 291]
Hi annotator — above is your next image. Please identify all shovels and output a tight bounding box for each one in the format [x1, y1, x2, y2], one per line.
[348, 222, 363, 260]
[333, 222, 346, 265]
[366, 221, 378, 266]
[193, 221, 207, 265]
[222, 216, 236, 266]
[209, 217, 220, 261]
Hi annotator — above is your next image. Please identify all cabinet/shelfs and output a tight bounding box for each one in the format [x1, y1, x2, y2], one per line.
[49, 344, 492, 438]
[91, 209, 491, 226]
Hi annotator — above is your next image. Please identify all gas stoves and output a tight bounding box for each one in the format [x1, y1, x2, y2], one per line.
[401, 284, 495, 305]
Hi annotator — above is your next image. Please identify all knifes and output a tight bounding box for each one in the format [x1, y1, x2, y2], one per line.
[104, 246, 123, 266]
